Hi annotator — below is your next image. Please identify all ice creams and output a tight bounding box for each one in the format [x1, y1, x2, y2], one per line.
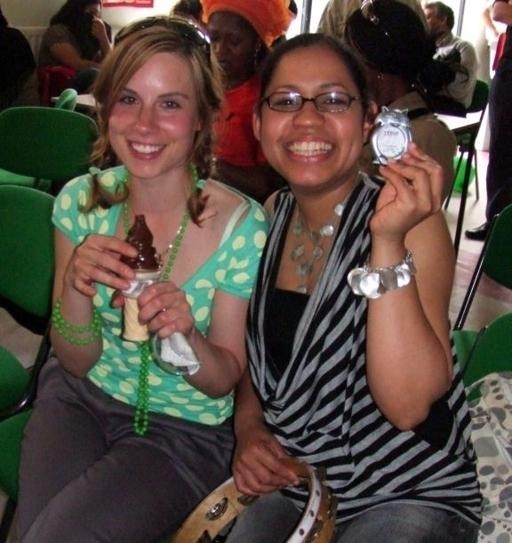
[118, 214, 163, 341]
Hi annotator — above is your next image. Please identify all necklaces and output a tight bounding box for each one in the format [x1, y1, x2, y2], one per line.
[290, 191, 353, 294]
[109, 160, 204, 437]
[151, 228, 177, 262]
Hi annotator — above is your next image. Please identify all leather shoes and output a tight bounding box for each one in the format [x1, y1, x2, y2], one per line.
[464, 221, 491, 241]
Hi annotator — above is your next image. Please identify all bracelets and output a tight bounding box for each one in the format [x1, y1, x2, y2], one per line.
[54, 295, 100, 333]
[49, 310, 103, 350]
[344, 242, 420, 302]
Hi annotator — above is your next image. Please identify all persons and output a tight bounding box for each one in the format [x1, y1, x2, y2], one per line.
[7, 15, 269, 542]
[219, 31, 484, 541]
[1, 0, 512, 240]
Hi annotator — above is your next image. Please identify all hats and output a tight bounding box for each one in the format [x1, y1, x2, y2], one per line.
[199, 0, 295, 49]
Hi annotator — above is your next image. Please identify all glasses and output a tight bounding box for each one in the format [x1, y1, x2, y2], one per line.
[260, 90, 361, 113]
[113, 13, 212, 46]
[361, 0, 390, 37]
